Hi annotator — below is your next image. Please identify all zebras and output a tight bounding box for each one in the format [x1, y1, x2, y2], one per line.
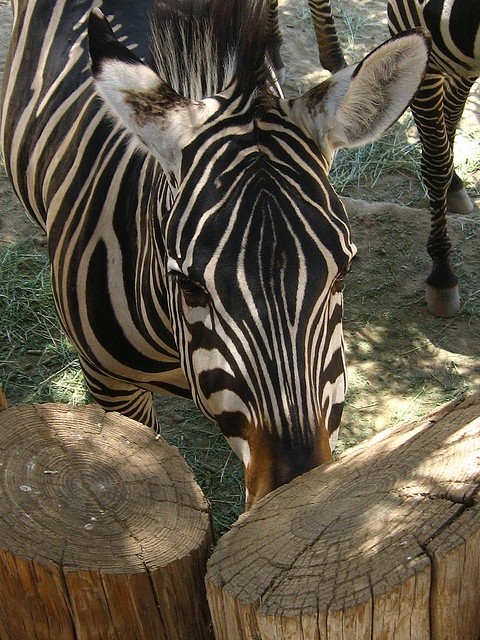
[266, 0, 480, 320]
[0, 0, 435, 516]
